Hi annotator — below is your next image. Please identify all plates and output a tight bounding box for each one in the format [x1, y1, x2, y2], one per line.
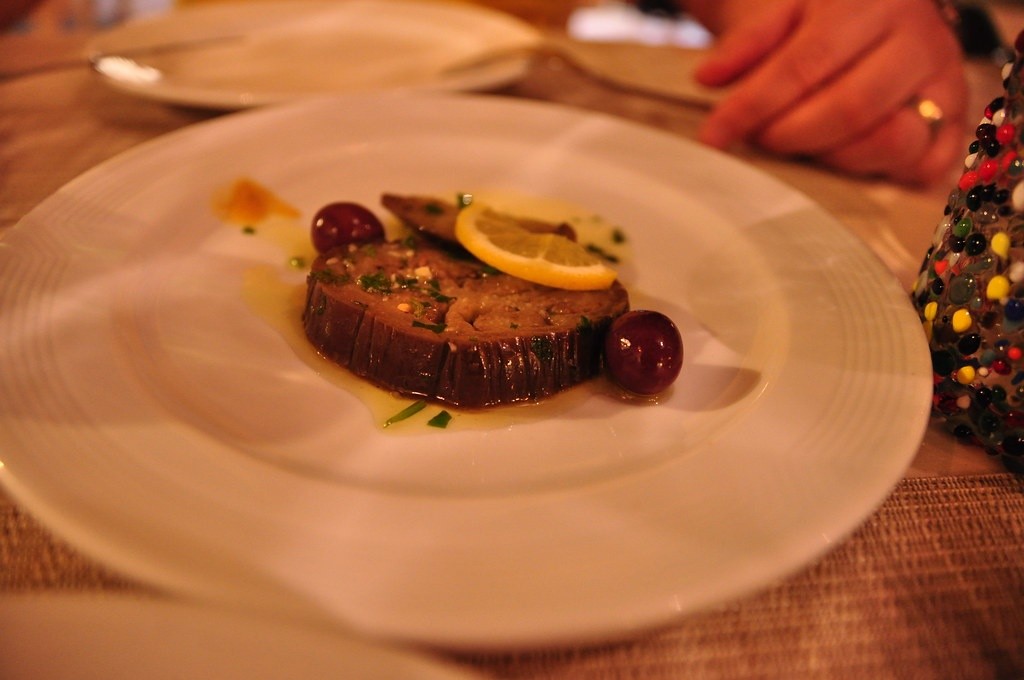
[1, 595, 482, 678]
[84, 1, 541, 109]
[0, 93, 931, 649]
[561, 3, 723, 109]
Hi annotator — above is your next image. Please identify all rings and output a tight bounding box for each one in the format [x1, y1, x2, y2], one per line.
[908, 96, 942, 142]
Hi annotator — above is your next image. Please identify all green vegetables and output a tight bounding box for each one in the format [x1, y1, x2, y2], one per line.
[307, 204, 596, 428]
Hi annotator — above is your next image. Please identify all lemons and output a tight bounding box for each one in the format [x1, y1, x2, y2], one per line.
[457, 200, 618, 291]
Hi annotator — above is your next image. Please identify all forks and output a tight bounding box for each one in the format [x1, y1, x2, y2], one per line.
[440, 44, 717, 115]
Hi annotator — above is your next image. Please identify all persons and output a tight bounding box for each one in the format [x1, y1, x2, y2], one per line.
[635, 0, 969, 185]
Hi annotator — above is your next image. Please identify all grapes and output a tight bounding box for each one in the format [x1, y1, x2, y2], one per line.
[606, 308, 683, 395]
[310, 202, 384, 252]
